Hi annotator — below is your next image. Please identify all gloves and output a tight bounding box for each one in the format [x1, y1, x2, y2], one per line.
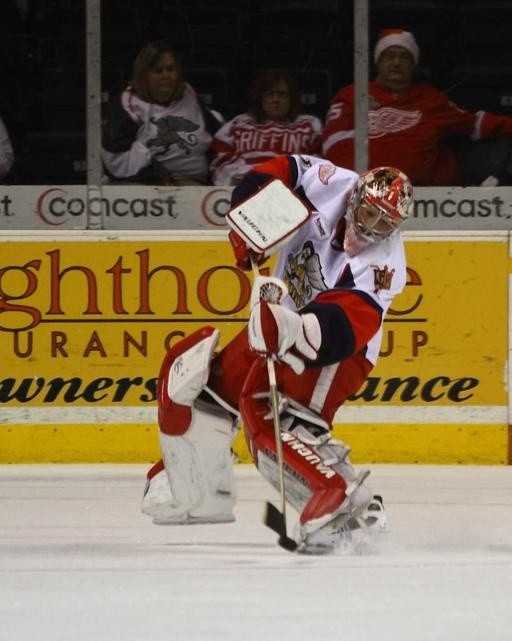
[247, 303, 321, 376]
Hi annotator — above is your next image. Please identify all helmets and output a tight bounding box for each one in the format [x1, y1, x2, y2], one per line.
[344, 167, 414, 253]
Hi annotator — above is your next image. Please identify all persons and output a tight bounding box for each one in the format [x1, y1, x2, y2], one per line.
[139, 151, 414, 558]
[207, 63, 324, 190]
[101, 40, 226, 189]
[320, 26, 510, 189]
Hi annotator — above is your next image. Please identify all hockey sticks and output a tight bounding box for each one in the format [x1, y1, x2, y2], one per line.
[247, 252, 286, 542]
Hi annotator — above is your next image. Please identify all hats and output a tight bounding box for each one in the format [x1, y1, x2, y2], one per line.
[372, 30, 418, 64]
